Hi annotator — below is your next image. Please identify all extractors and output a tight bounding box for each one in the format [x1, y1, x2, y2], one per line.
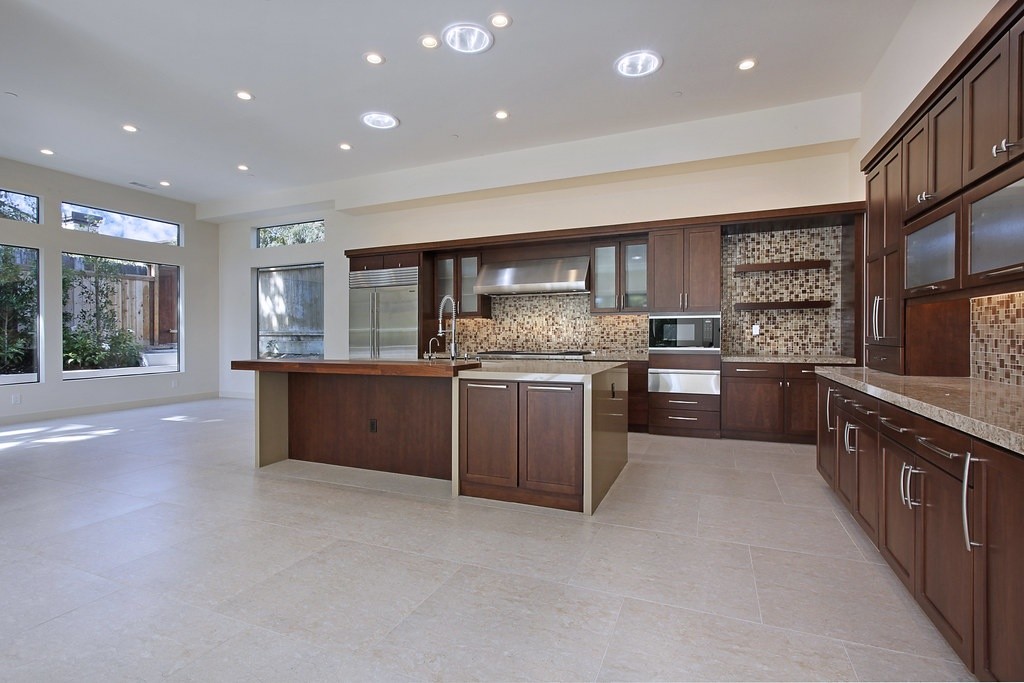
[473, 255, 591, 297]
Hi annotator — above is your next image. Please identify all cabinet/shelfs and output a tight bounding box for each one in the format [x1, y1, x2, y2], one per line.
[815, 375, 835, 493]
[384, 244, 420, 268]
[590, 222, 649, 313]
[961, 438, 1024, 682]
[720, 363, 856, 444]
[902, 46, 961, 222]
[648, 353, 721, 439]
[960, 162, 1024, 286]
[902, 197, 961, 297]
[457, 380, 583, 513]
[649, 215, 721, 312]
[431, 237, 481, 317]
[836, 383, 879, 552]
[861, 126, 905, 376]
[344, 247, 384, 271]
[880, 401, 974, 673]
[960, 15, 1023, 188]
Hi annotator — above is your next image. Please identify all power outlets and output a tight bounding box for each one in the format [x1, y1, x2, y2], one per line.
[753, 325, 759, 335]
[369, 419, 377, 432]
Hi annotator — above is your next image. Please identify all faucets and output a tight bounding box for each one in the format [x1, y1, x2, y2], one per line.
[428, 337, 439, 362]
[438, 296, 457, 359]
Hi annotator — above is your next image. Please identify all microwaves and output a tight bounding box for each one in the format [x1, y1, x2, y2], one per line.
[648, 315, 721, 350]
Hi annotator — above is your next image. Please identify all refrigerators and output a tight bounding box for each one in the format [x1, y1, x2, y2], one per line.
[349, 267, 419, 360]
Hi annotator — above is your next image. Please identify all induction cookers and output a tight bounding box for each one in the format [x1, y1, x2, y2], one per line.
[477, 348, 591, 361]
